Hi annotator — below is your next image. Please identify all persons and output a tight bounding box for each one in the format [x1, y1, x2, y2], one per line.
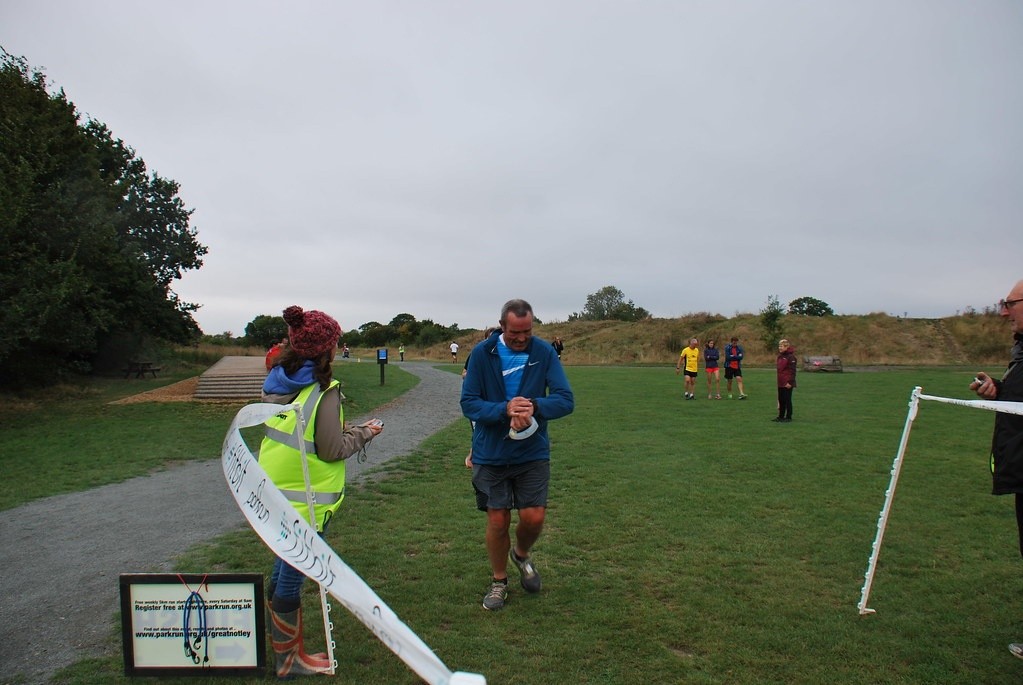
[449, 340, 460, 362]
[969, 279, 1023, 659]
[461, 299, 575, 611]
[253, 305, 383, 679]
[676, 337, 701, 401]
[771, 339, 797, 423]
[704, 338, 723, 400]
[724, 337, 748, 400]
[399, 343, 405, 361]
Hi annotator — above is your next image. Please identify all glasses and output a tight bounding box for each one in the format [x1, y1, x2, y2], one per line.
[999, 298, 1022, 310]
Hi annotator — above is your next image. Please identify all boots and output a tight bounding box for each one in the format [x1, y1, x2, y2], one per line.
[266, 599, 330, 680]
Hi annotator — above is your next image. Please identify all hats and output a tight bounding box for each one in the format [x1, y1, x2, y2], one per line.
[282, 304, 341, 357]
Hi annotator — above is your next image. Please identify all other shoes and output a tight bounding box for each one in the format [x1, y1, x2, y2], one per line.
[683, 392, 748, 401]
[770, 417, 792, 423]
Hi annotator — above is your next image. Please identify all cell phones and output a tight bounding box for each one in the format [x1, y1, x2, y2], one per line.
[370, 419, 384, 428]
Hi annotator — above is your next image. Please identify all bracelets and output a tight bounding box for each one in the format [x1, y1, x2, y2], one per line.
[676, 367, 680, 369]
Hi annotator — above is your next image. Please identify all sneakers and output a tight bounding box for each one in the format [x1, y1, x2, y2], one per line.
[508, 548, 540, 593]
[482, 582, 507, 612]
